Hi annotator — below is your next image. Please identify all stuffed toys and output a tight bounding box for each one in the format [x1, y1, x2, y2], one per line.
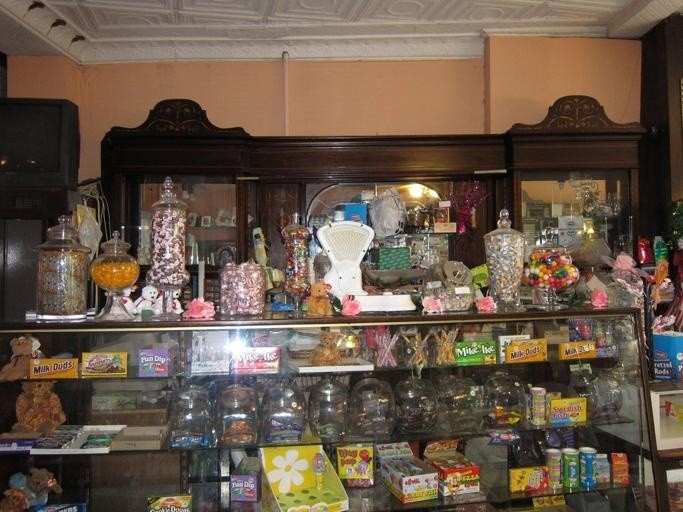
[133, 285, 160, 314]
[154, 287, 184, 314]
[107, 286, 137, 315]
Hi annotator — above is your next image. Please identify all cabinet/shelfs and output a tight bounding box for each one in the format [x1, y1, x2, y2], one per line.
[97, 99, 646, 300]
[1, 101, 86, 298]
[0, 308, 670, 512]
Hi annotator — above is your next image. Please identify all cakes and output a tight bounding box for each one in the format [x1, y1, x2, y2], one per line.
[219, 259, 266, 315]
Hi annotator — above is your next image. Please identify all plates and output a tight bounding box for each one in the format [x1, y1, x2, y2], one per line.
[213, 244, 236, 266]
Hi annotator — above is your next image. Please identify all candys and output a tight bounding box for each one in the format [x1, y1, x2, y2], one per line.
[281, 226, 310, 295]
[90, 260, 140, 289]
[439, 289, 473, 311]
[486, 233, 579, 293]
[145, 208, 191, 286]
[36, 251, 89, 315]
[372, 327, 460, 369]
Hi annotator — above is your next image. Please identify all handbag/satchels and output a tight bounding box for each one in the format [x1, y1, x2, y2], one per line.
[367, 187, 405, 240]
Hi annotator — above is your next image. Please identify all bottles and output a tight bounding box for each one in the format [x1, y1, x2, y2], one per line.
[573, 371, 627, 420]
[219, 384, 259, 450]
[261, 385, 306, 444]
[484, 372, 527, 430]
[171, 385, 218, 454]
[308, 382, 352, 443]
[441, 373, 482, 436]
[354, 379, 396, 443]
[398, 375, 439, 438]
